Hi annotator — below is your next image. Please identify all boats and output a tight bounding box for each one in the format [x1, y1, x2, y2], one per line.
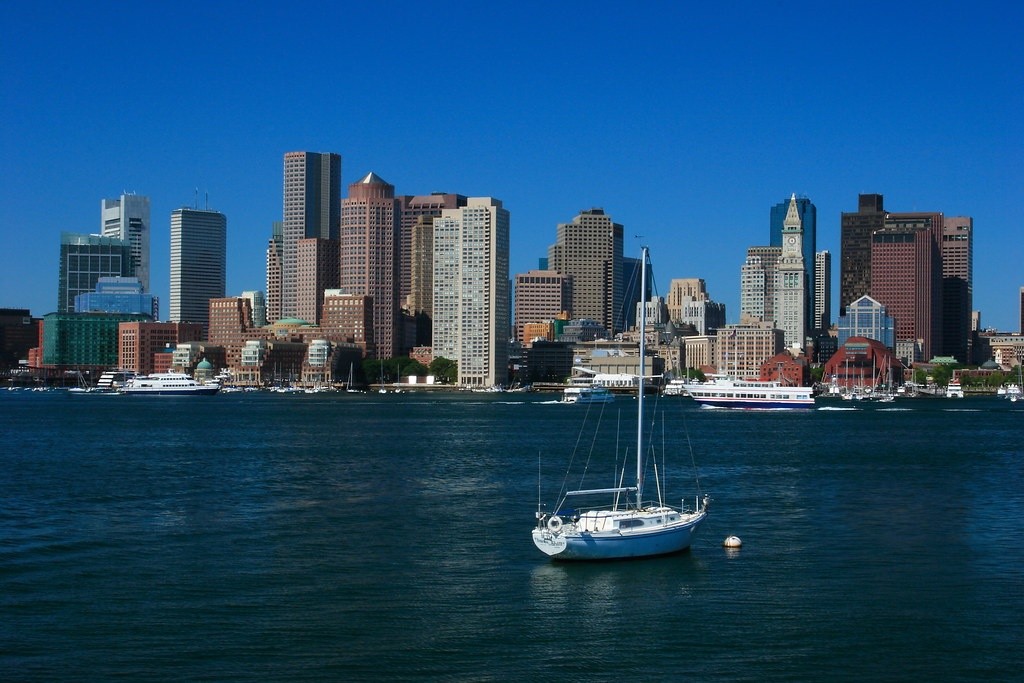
[116, 372, 221, 396]
[841, 356, 897, 403]
[562, 387, 616, 403]
[947, 383, 965, 399]
[684, 381, 816, 409]
[997, 384, 1023, 402]
[532, 246, 709, 559]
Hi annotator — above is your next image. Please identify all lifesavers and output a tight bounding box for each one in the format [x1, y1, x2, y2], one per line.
[548, 516, 563, 533]
